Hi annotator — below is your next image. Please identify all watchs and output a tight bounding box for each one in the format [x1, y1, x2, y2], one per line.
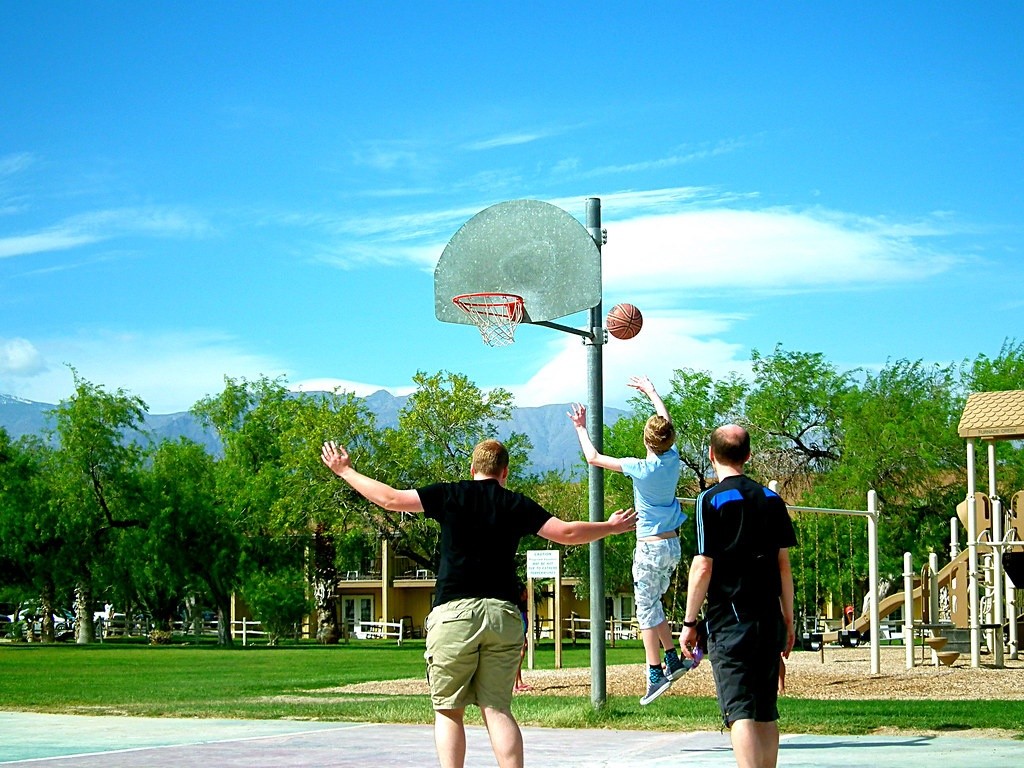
[684, 617, 698, 628]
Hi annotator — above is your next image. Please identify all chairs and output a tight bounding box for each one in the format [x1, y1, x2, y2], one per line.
[403, 615, 422, 639]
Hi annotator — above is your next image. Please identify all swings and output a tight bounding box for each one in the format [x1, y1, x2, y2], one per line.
[832, 514, 861, 650]
[796, 511, 824, 652]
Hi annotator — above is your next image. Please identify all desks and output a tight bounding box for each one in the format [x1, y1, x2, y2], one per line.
[346, 571, 360, 581]
[416, 570, 434, 580]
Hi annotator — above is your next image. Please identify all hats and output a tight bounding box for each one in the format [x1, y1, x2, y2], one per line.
[845, 606, 853, 614]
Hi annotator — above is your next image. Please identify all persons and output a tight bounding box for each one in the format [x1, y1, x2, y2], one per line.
[567, 374, 687, 705]
[679, 424, 796, 768]
[320, 438, 639, 768]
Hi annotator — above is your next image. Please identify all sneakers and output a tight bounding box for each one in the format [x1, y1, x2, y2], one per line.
[639, 677, 671, 705]
[664, 663, 686, 681]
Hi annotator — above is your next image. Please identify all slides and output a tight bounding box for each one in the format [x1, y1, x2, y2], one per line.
[823, 490, 993, 644]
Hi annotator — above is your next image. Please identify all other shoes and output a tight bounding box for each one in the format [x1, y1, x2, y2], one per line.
[777, 690, 785, 698]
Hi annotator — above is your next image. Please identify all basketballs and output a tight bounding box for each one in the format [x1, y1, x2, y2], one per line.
[606, 302, 644, 342]
